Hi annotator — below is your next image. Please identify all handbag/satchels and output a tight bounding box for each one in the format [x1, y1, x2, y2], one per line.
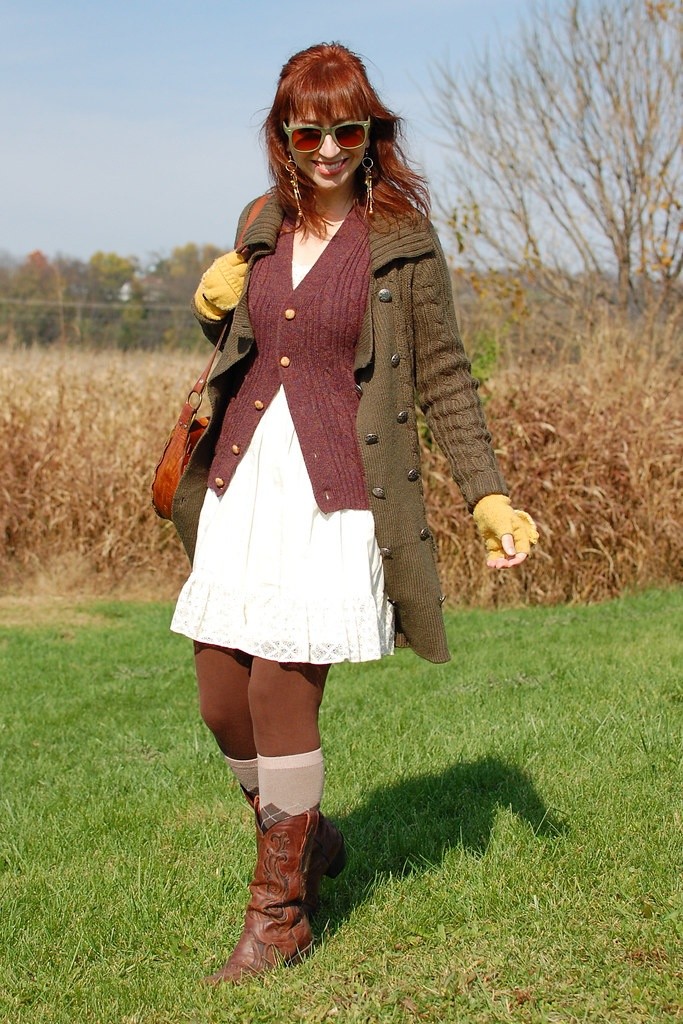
[151, 404, 211, 521]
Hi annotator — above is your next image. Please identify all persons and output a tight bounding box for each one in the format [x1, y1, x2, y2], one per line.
[167, 43, 540, 987]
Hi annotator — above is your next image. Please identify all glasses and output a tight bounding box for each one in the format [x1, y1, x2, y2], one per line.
[282, 115, 370, 153]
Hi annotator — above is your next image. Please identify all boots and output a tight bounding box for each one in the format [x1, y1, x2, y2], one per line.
[201, 782, 346, 987]
